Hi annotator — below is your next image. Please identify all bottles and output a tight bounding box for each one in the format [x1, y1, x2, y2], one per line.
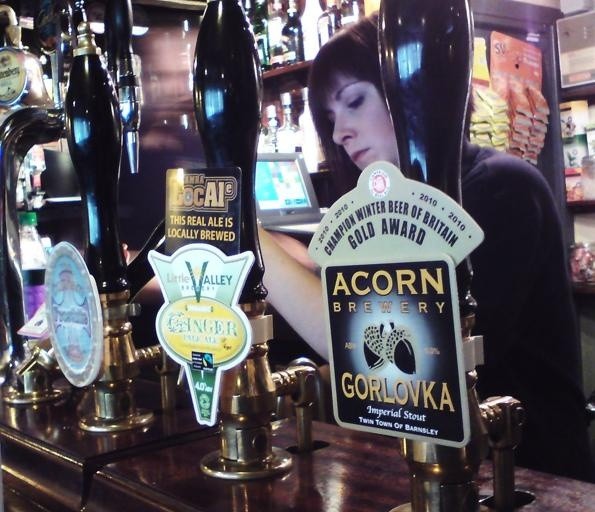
[245, 0, 329, 69]
[190, 0, 269, 301]
[260, 87, 320, 173]
[374, 1, 477, 315]
[62, 1, 143, 291]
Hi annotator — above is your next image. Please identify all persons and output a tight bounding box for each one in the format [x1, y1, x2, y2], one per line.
[257, 9, 595, 482]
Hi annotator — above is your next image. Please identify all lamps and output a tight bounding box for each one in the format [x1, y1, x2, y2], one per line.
[1, 3, 55, 108]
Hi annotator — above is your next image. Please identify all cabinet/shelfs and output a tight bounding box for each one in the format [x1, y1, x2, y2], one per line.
[242, 0, 380, 177]
[554, 23, 595, 287]
[465, 0, 568, 272]
